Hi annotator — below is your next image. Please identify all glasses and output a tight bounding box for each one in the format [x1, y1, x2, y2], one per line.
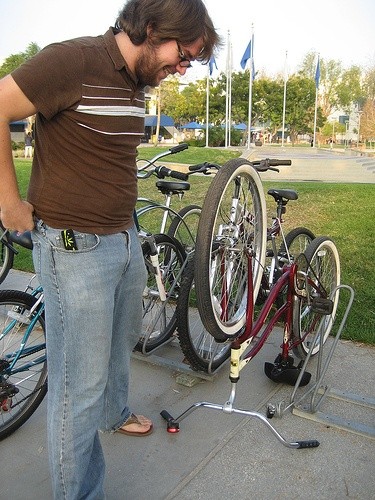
[175, 38, 193, 69]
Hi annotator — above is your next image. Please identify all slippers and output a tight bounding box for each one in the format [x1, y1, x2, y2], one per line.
[112, 414, 154, 437]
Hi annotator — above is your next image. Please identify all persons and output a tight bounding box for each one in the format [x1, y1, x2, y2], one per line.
[1, 0, 228, 500]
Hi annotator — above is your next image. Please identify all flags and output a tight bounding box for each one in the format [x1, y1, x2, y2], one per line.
[207, 50, 216, 73]
[314, 58, 322, 88]
[240, 39, 254, 70]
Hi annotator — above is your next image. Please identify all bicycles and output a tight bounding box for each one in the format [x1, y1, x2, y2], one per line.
[160, 153, 356, 449]
[0, 142, 203, 443]
[176, 153, 324, 378]
